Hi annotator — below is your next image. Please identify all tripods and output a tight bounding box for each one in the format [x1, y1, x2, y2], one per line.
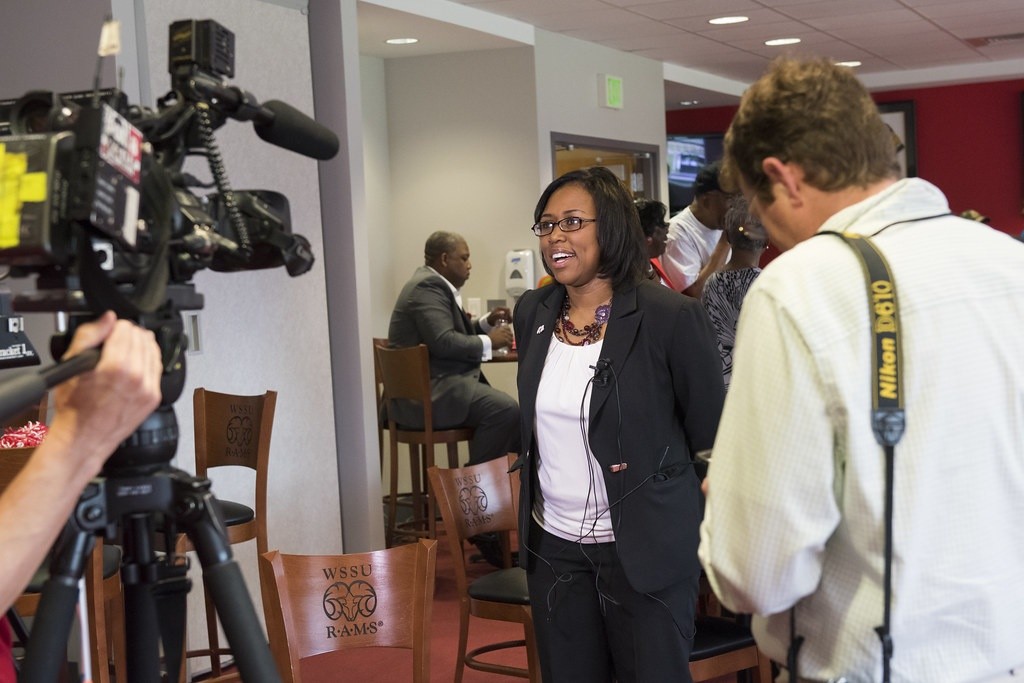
[0, 282, 289, 683]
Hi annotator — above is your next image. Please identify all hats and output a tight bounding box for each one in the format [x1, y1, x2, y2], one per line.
[961, 210, 990, 224]
[694, 160, 723, 195]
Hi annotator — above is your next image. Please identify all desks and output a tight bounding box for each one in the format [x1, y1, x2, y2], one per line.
[481, 350, 518, 363]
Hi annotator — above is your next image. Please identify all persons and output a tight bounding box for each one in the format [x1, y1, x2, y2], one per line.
[632, 197, 682, 292]
[379, 231, 519, 573]
[0, 308, 163, 627]
[659, 161, 733, 299]
[698, 54, 1024, 683]
[701, 194, 770, 390]
[511, 165, 726, 683]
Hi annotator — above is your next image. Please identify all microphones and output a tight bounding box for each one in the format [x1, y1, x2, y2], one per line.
[601, 369, 609, 382]
[196, 79, 340, 161]
[597, 359, 611, 370]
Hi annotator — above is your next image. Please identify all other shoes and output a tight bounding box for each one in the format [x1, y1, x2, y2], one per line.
[468, 532, 517, 569]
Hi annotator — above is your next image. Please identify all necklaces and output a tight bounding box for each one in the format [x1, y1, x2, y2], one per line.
[647, 261, 656, 280]
[555, 293, 611, 346]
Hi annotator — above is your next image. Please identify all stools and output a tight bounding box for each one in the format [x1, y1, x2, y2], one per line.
[426, 452, 543, 683]
[1, 387, 278, 683]
[260, 538, 439, 683]
[689, 614, 772, 683]
[373, 338, 477, 549]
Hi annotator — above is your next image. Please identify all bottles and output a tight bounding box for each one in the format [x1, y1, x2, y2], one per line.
[494, 310, 510, 356]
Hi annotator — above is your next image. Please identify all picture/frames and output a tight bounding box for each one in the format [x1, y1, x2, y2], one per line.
[876, 99, 916, 179]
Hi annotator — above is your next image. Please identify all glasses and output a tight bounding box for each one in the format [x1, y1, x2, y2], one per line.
[532, 217, 596, 236]
[738, 175, 769, 241]
[654, 222, 670, 230]
